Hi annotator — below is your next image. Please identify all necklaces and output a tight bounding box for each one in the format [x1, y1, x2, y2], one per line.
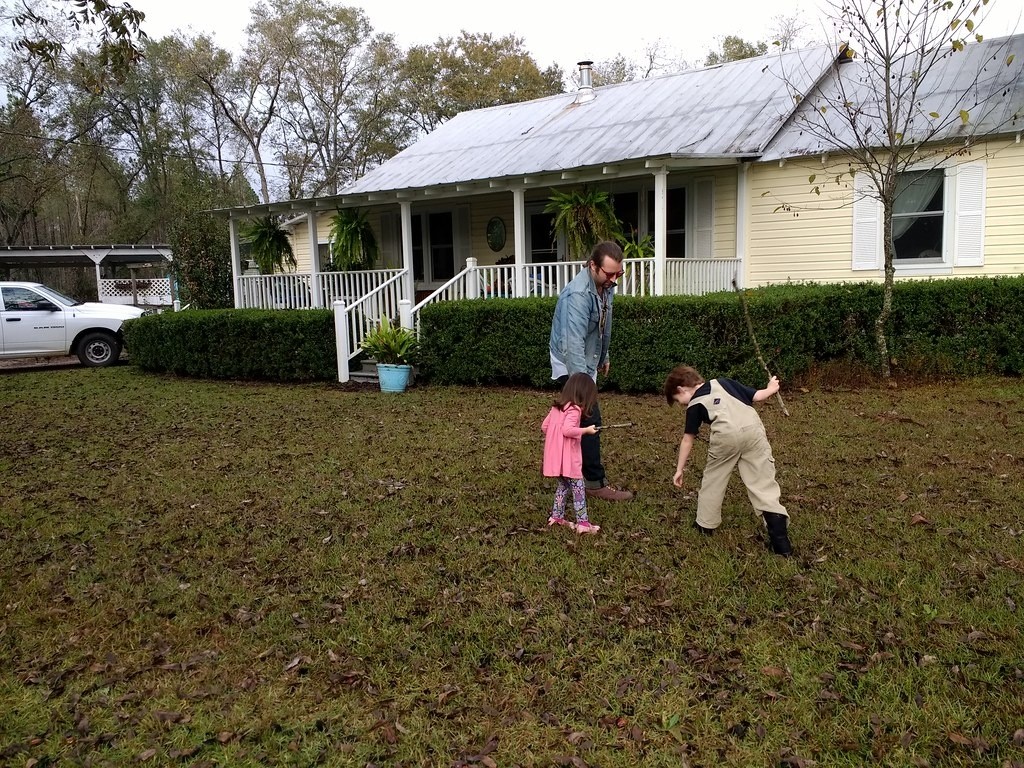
[601, 292, 606, 312]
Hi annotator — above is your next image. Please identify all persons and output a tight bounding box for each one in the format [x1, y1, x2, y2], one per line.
[541, 372, 602, 536]
[549, 241, 634, 500]
[662, 365, 795, 558]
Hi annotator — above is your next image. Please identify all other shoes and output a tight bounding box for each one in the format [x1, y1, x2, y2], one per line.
[548, 516, 575, 529]
[575, 522, 600, 534]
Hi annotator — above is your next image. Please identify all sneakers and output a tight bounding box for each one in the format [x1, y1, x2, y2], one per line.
[586, 484, 633, 500]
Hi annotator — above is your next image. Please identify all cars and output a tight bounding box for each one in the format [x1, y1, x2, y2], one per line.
[0, 278, 149, 367]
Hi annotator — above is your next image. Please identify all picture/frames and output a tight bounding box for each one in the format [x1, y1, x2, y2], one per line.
[486, 216, 506, 253]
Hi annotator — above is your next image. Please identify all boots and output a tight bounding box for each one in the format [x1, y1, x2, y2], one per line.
[756, 511, 793, 558]
[692, 521, 714, 537]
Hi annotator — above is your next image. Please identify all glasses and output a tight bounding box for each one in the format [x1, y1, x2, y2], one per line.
[601, 267, 624, 279]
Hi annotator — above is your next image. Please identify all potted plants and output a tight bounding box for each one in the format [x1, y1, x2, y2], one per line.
[357, 312, 422, 393]
